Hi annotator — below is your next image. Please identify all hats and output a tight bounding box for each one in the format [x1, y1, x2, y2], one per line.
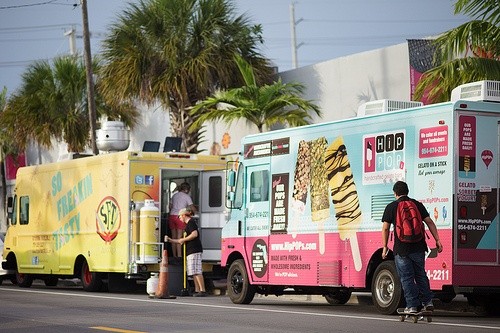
[176, 208, 191, 218]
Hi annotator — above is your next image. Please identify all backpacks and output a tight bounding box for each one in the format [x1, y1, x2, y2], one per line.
[394, 198, 425, 242]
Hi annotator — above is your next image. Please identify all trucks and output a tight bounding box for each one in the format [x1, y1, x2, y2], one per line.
[2, 151, 235, 294]
[221, 81, 500, 316]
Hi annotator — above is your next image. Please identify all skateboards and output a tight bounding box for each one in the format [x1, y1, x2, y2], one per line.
[397, 306, 434, 324]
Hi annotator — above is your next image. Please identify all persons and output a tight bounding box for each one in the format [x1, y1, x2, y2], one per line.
[164, 207, 210, 299]
[168, 182, 198, 260]
[381, 179, 443, 315]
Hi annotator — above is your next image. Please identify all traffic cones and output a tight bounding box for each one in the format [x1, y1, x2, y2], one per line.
[148, 250, 177, 299]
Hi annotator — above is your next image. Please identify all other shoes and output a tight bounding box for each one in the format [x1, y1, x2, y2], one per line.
[189, 291, 207, 297]
[421, 301, 433, 313]
[404, 307, 417, 313]
